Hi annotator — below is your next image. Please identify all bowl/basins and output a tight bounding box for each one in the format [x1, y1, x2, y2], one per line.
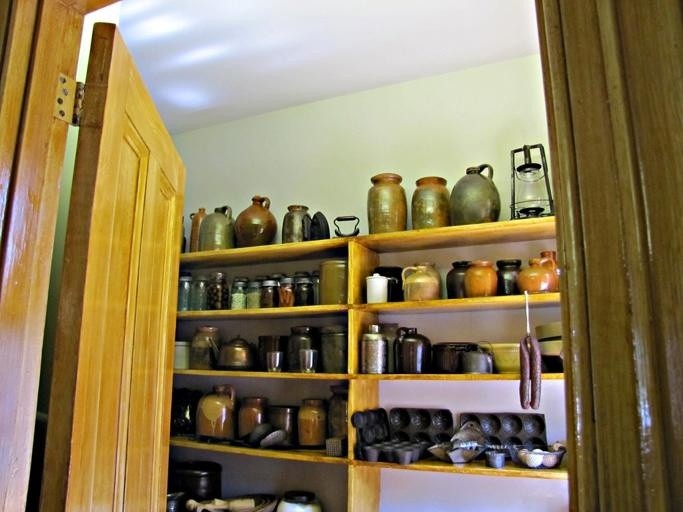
[535, 320, 567, 373]
[506, 444, 565, 469]
[479, 342, 521, 374]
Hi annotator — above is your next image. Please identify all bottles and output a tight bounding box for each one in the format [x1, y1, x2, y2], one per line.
[281, 204, 310, 244]
[410, 177, 450, 229]
[173, 325, 220, 370]
[360, 323, 388, 374]
[199, 206, 235, 252]
[364, 273, 389, 304]
[190, 208, 205, 252]
[177, 268, 319, 311]
[449, 163, 502, 223]
[366, 172, 408, 234]
[178, 383, 350, 452]
[374, 252, 565, 304]
[234, 194, 277, 247]
[376, 322, 435, 373]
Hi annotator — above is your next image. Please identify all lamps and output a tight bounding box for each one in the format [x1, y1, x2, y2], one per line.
[507, 142, 555, 219]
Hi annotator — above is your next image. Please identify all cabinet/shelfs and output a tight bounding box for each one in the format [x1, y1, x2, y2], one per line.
[166, 214, 568, 511]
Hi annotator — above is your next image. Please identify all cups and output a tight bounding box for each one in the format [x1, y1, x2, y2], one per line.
[362, 437, 430, 466]
[297, 349, 318, 373]
[265, 351, 283, 371]
[485, 451, 506, 467]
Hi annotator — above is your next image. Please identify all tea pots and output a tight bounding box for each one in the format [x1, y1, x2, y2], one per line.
[459, 339, 494, 375]
[206, 334, 255, 370]
